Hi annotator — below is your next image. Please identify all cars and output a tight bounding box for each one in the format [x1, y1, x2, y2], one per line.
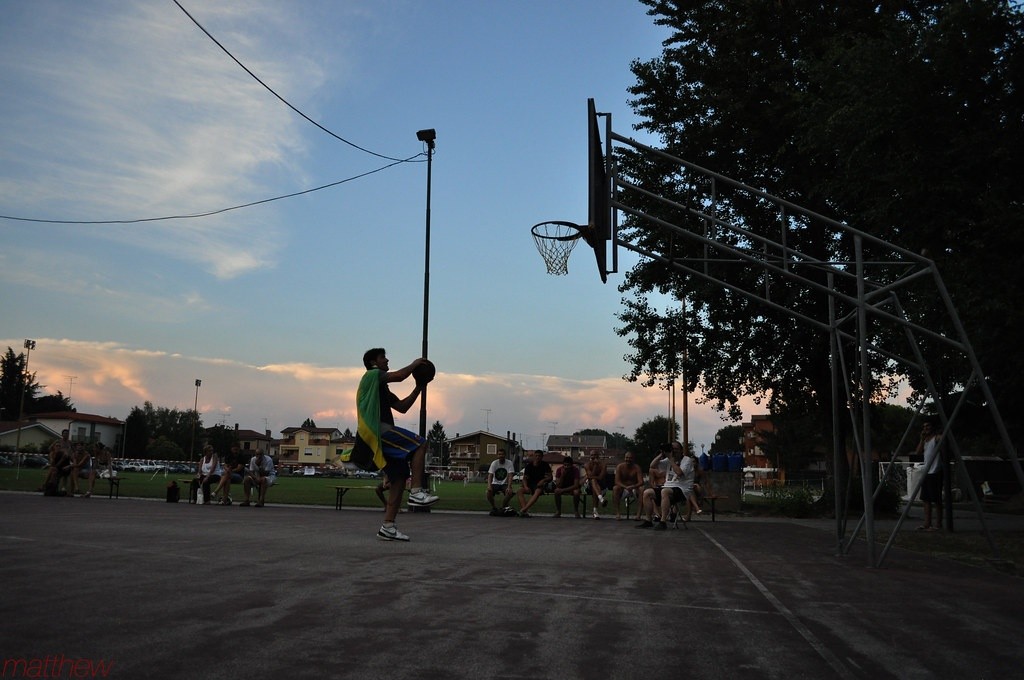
[0, 456, 49, 466]
[110, 461, 152, 472]
[428, 470, 443, 480]
[143, 460, 190, 472]
[324, 469, 348, 477]
[353, 470, 377, 478]
[293, 468, 323, 477]
[449, 471, 467, 481]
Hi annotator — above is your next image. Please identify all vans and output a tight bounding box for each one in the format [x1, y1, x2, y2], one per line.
[512, 469, 524, 483]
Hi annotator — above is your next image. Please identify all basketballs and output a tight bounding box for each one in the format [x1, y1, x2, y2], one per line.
[412, 360, 436, 383]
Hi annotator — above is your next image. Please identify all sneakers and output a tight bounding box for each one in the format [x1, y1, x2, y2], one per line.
[375, 525, 410, 542]
[406, 488, 440, 507]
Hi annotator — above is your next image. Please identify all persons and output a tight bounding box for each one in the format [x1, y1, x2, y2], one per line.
[583, 450, 609, 520]
[33, 429, 114, 498]
[349, 348, 440, 543]
[191, 445, 221, 504]
[612, 452, 645, 521]
[239, 447, 276, 508]
[376, 473, 404, 513]
[487, 449, 516, 513]
[913, 417, 948, 532]
[554, 456, 583, 519]
[517, 449, 554, 517]
[211, 443, 246, 505]
[635, 441, 715, 531]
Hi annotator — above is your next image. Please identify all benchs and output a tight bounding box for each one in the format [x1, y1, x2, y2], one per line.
[68, 473, 127, 500]
[177, 478, 279, 503]
[331, 485, 414, 512]
[624, 494, 729, 522]
[495, 489, 591, 518]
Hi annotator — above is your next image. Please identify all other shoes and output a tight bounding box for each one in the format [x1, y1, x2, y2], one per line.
[489, 509, 497, 515]
[670, 510, 677, 522]
[575, 513, 581, 518]
[635, 521, 652, 528]
[593, 512, 599, 519]
[602, 499, 608, 508]
[240, 500, 251, 506]
[653, 521, 667, 531]
[654, 517, 662, 521]
[255, 502, 263, 507]
[553, 513, 561, 517]
[84, 492, 90, 496]
[697, 510, 702, 515]
[634, 517, 641, 521]
[682, 515, 690, 522]
[211, 493, 215, 497]
[916, 523, 940, 532]
[519, 511, 528, 517]
[616, 513, 621, 520]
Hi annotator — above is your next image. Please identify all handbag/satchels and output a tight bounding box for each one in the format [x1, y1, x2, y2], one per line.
[218, 496, 232, 505]
[196, 488, 204, 504]
[498, 507, 519, 517]
[45, 482, 58, 496]
[167, 482, 180, 503]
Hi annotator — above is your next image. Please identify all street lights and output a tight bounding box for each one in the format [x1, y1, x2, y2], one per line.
[14, 339, 36, 466]
[190, 379, 202, 469]
